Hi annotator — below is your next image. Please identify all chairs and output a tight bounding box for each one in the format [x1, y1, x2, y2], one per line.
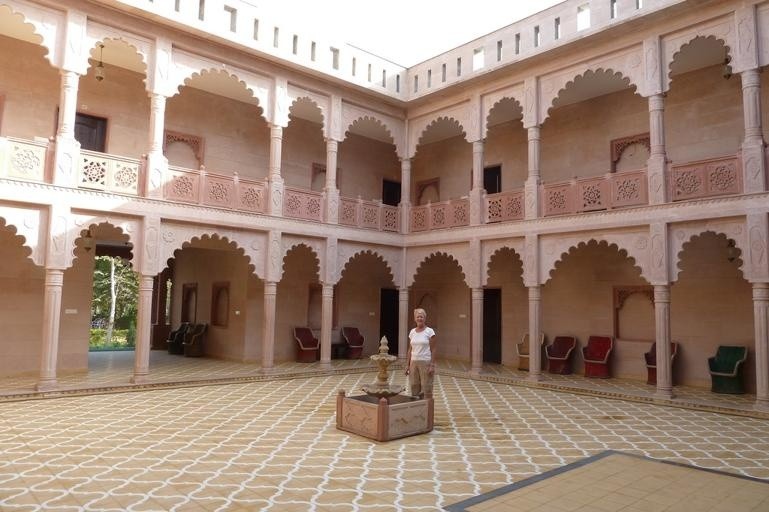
[293, 326, 319, 363]
[341, 326, 365, 359]
[515, 331, 544, 371]
[707, 345, 748, 395]
[544, 336, 577, 375]
[166, 322, 192, 354]
[181, 323, 208, 358]
[642, 341, 678, 386]
[580, 336, 613, 380]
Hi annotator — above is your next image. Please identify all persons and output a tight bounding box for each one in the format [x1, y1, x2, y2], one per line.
[404, 307, 437, 398]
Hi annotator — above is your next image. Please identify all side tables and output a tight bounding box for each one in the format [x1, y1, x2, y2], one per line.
[319, 343, 344, 359]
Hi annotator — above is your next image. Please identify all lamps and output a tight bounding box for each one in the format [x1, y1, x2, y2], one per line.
[719, 47, 731, 80]
[725, 239, 736, 263]
[95, 45, 105, 82]
